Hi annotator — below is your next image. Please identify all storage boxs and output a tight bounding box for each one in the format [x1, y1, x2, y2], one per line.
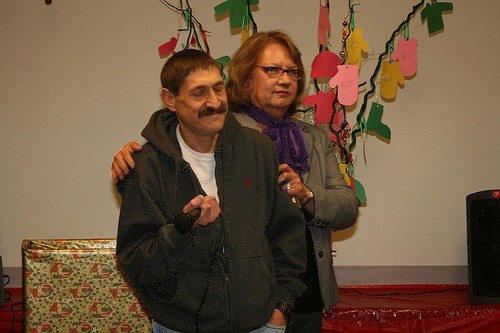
[20, 237, 153, 333]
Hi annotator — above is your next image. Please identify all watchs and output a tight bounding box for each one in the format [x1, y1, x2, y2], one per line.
[301, 183, 314, 206]
[276, 301, 294, 322]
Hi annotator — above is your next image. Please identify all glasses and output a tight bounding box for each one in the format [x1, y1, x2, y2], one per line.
[255, 64, 303, 80]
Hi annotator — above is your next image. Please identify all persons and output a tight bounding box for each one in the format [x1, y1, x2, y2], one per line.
[110, 30, 362, 333]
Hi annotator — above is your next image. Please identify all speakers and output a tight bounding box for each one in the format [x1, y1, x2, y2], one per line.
[467, 190, 500, 304]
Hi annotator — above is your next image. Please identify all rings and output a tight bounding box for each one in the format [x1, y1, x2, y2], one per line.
[285, 182, 291, 190]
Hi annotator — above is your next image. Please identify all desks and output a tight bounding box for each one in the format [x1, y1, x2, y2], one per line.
[0, 266, 500, 333]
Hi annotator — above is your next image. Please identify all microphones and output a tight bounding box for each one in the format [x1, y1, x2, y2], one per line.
[174, 208, 201, 235]
